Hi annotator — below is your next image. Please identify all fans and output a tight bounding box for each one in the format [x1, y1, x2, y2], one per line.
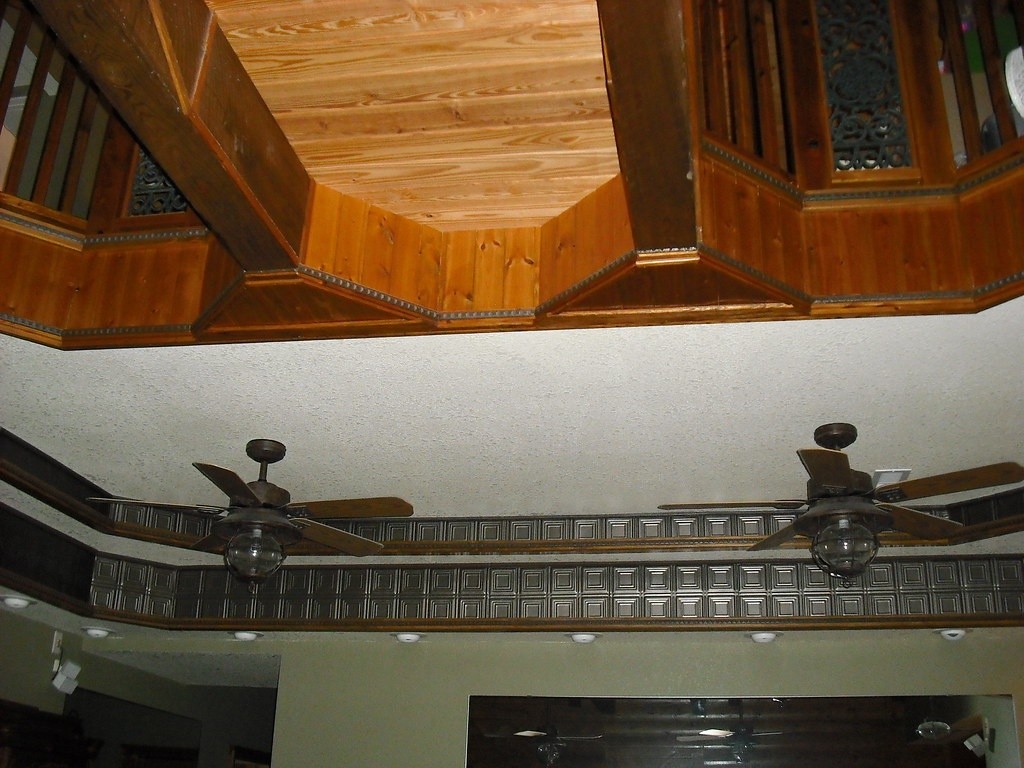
[677, 713, 786, 742]
[657, 422, 1024, 551]
[483, 703, 604, 742]
[87, 439, 414, 556]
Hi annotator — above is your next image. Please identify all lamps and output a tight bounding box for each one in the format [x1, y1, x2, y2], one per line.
[931, 629, 974, 640]
[791, 496, 895, 591]
[227, 632, 264, 641]
[527, 737, 568, 766]
[81, 626, 116, 640]
[564, 632, 604, 644]
[209, 509, 303, 593]
[389, 633, 427, 643]
[725, 735, 762, 764]
[0, 596, 39, 609]
[963, 717, 990, 757]
[51, 630, 81, 694]
[744, 631, 784, 643]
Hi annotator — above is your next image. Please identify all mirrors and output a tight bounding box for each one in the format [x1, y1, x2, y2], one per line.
[465, 694, 1022, 768]
